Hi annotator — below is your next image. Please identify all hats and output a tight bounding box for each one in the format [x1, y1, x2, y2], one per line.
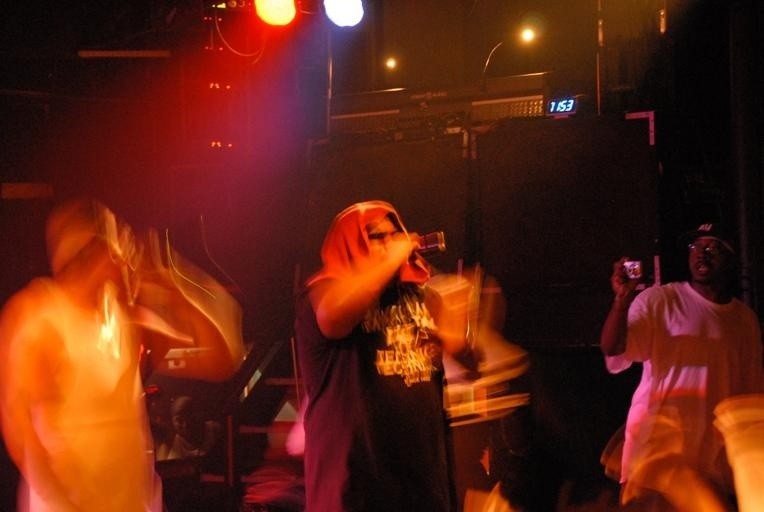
[690, 221, 740, 256]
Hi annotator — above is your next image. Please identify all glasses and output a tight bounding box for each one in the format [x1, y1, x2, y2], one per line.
[687, 241, 722, 256]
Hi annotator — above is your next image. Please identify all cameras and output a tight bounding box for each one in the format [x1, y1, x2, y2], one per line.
[623, 260, 642, 280]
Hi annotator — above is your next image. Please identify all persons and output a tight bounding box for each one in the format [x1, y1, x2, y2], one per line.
[596, 214, 763, 512]
[151, 392, 235, 512]
[284, 196, 489, 509]
[0, 190, 250, 510]
